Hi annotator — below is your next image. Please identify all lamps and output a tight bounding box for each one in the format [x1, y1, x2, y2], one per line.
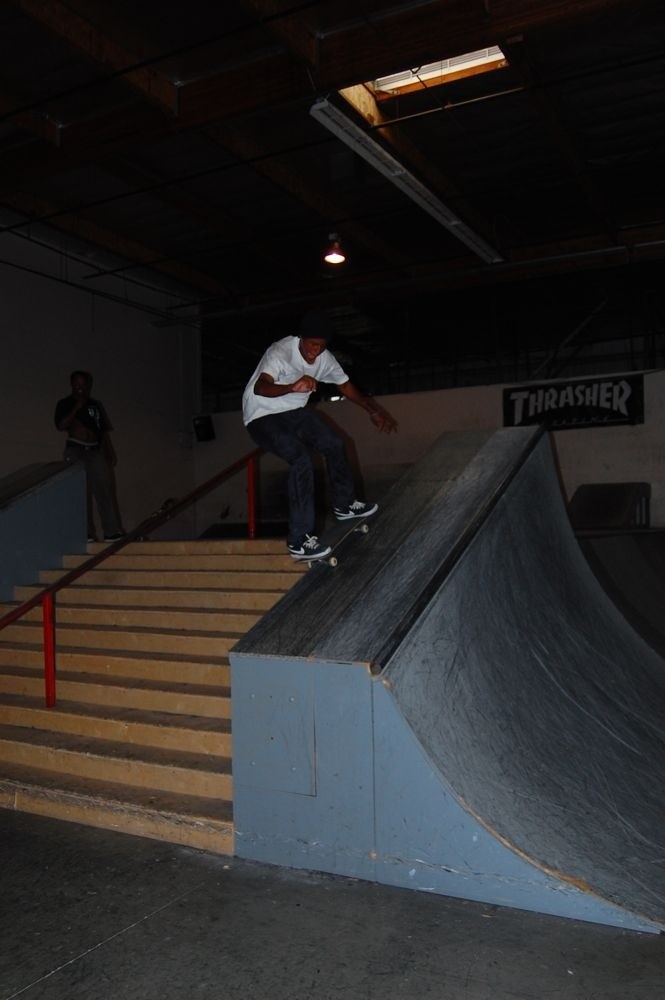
[322, 232, 347, 264]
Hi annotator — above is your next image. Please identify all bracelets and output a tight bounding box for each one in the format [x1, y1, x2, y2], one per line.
[369, 409, 382, 416]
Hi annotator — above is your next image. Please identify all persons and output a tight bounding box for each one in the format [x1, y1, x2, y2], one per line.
[53, 371, 127, 543]
[242, 320, 403, 559]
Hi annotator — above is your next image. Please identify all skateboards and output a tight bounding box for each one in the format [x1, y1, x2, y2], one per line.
[293, 516, 370, 566]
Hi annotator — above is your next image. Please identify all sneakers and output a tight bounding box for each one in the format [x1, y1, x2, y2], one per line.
[287, 534, 332, 560]
[333, 499, 379, 520]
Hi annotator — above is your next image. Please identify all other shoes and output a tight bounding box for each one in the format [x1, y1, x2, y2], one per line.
[88, 536, 94, 542]
[104, 534, 125, 542]
